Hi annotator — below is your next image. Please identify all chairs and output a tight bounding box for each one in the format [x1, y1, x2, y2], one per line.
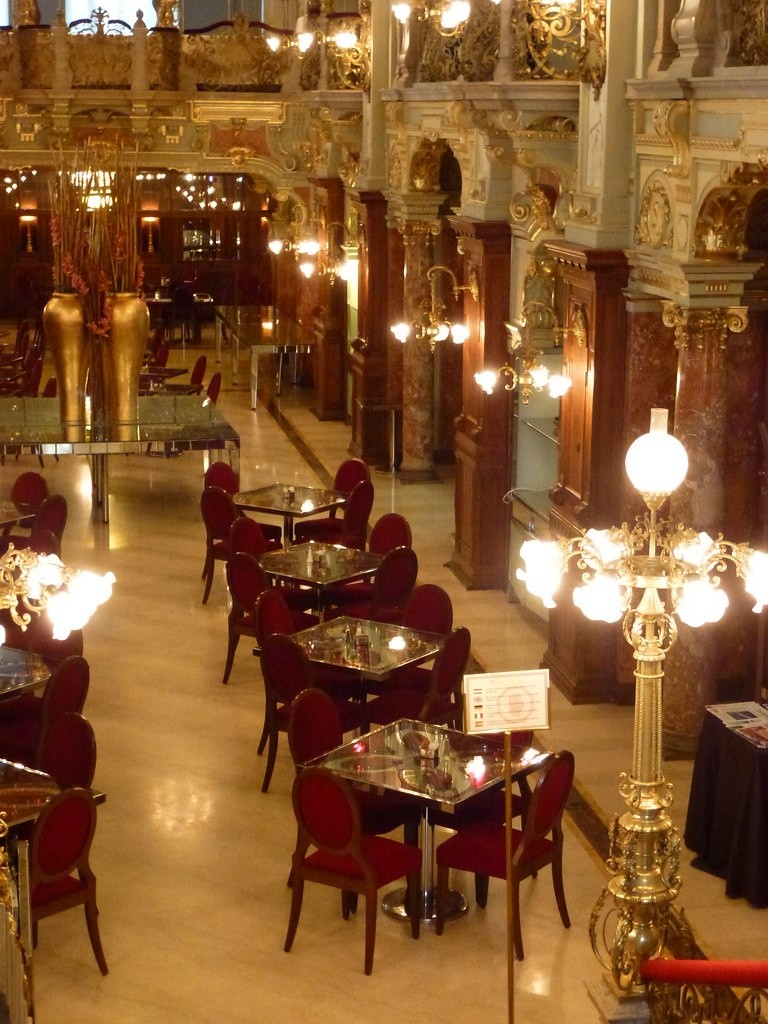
[200, 459, 576, 976]
[0, 274, 230, 468]
[0, 471, 110, 977]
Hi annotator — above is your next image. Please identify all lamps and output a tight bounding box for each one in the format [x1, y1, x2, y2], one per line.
[265, 0, 322, 91]
[474, 298, 588, 407]
[0, 542, 118, 648]
[392, 0, 470, 37]
[267, 205, 327, 261]
[297, 0, 371, 103]
[515, 407, 768, 660]
[298, 222, 362, 287]
[390, 263, 481, 353]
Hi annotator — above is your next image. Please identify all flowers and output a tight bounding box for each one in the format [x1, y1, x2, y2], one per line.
[43, 134, 147, 336]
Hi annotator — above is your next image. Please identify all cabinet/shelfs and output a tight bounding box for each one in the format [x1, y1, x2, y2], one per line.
[509, 417, 560, 623]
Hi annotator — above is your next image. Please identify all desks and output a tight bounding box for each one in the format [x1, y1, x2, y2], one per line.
[145, 290, 214, 344]
[253, 616, 449, 740]
[0, 645, 63, 701]
[0, 758, 106, 870]
[139, 384, 203, 396]
[214, 303, 316, 411]
[355, 397, 403, 475]
[258, 541, 385, 657]
[0, 497, 41, 536]
[231, 484, 351, 548]
[0, 394, 240, 524]
[683, 712, 768, 910]
[140, 368, 189, 383]
[296, 718, 555, 926]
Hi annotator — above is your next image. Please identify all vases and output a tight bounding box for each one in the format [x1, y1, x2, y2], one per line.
[99, 291, 151, 442]
[41, 291, 89, 443]
